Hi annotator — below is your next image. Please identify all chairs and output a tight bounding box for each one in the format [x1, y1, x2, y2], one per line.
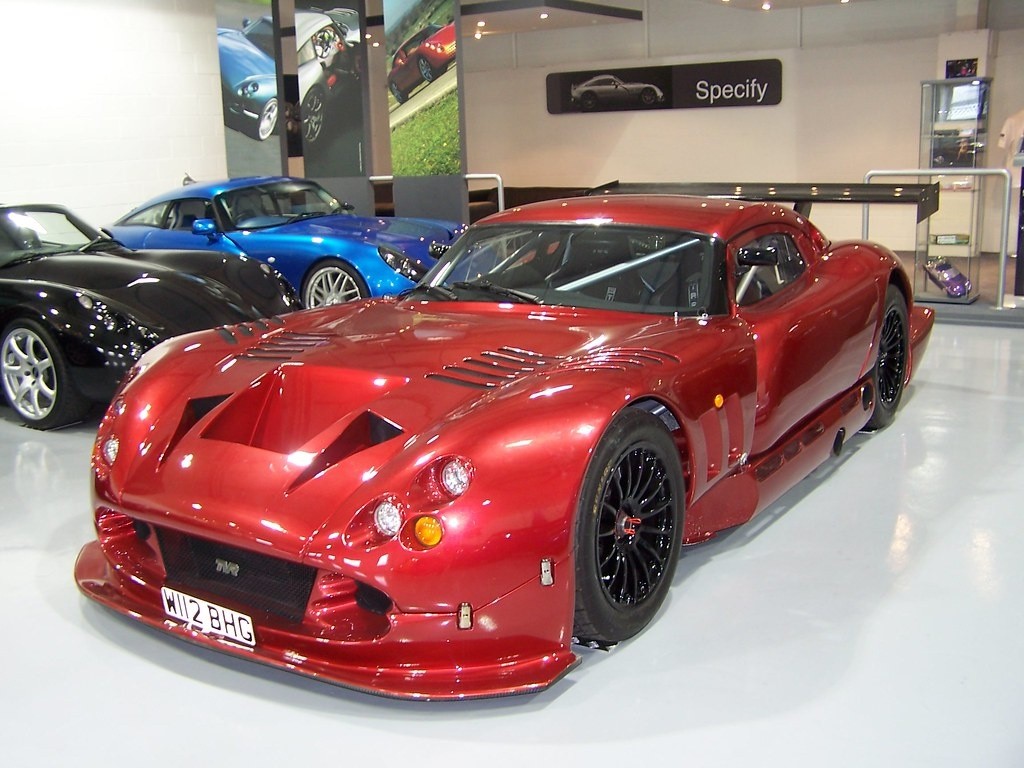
[546, 229, 642, 301]
[204, 203, 216, 219]
[654, 241, 766, 310]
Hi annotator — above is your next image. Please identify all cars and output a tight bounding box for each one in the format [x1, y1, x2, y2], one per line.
[217, 15, 278, 141]
[293, 8, 363, 148]
[387, 21, 456, 104]
[923, 255, 972, 299]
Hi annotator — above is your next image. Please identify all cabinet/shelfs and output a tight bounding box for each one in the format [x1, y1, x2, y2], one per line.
[912, 78, 994, 306]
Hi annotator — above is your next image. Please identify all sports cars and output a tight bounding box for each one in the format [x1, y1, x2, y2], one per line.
[0, 201, 300, 431]
[71, 193, 937, 706]
[106, 175, 501, 314]
[570, 74, 666, 112]
